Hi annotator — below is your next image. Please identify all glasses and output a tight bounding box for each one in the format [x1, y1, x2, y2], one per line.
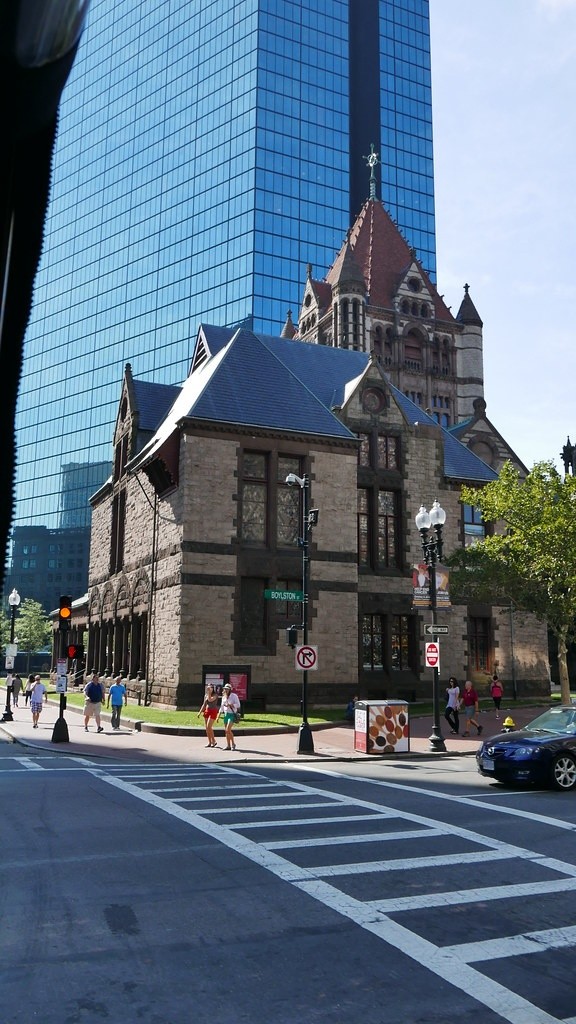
[450, 681, 453, 682]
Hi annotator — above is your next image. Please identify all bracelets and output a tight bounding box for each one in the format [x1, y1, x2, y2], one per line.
[103, 700, 105, 701]
[475, 710, 480, 712]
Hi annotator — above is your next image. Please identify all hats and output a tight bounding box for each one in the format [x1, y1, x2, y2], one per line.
[223, 683, 233, 690]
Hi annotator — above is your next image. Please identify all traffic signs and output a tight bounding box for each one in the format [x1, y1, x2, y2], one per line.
[424, 624, 449, 635]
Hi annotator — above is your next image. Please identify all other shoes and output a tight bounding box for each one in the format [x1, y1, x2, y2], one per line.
[231, 744, 236, 750]
[33, 723, 39, 728]
[496, 715, 500, 719]
[450, 728, 457, 734]
[223, 747, 231, 750]
[112, 726, 120, 731]
[205, 742, 217, 747]
[97, 727, 104, 733]
[462, 731, 471, 737]
[477, 725, 483, 735]
[85, 727, 89, 732]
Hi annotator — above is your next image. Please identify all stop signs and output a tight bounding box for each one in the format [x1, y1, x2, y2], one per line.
[425, 642, 440, 667]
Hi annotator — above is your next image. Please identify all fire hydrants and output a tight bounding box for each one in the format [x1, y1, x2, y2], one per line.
[500, 716, 515, 733]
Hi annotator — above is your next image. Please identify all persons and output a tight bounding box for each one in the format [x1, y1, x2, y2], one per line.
[30, 675, 48, 728]
[107, 677, 127, 731]
[490, 675, 503, 720]
[83, 675, 106, 733]
[457, 681, 484, 737]
[12, 674, 23, 708]
[197, 683, 218, 747]
[216, 683, 241, 751]
[345, 695, 361, 720]
[24, 674, 35, 706]
[5, 671, 13, 694]
[444, 677, 461, 736]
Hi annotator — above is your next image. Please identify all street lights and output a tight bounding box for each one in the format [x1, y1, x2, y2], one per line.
[415, 496, 447, 753]
[9, 636, 19, 691]
[1, 588, 20, 721]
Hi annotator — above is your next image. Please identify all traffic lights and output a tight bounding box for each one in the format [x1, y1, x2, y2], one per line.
[66, 645, 84, 659]
[59, 594, 71, 630]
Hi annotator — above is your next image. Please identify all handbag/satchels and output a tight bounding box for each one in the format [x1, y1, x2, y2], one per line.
[234, 713, 241, 724]
[440, 688, 449, 702]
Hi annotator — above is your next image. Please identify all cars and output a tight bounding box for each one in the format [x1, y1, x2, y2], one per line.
[476, 701, 576, 792]
